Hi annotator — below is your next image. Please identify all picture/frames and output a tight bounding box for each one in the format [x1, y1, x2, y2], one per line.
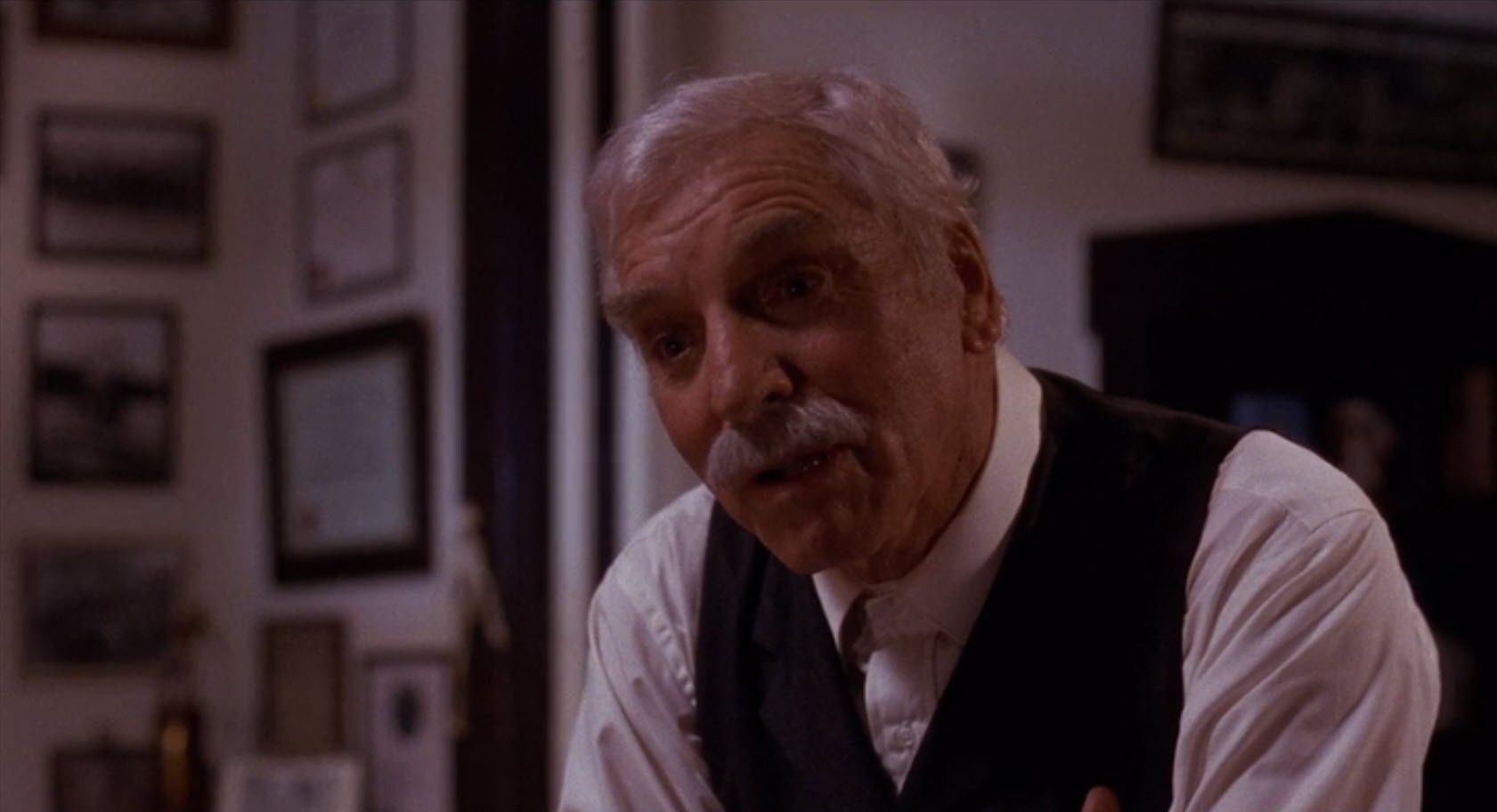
[18, 533, 191, 684]
[266, 313, 434, 588]
[21, 291, 181, 483]
[33, 103, 216, 264]
[30, 0, 235, 48]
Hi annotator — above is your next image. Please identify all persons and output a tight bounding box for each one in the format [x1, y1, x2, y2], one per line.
[558, 64, 1438, 812]
